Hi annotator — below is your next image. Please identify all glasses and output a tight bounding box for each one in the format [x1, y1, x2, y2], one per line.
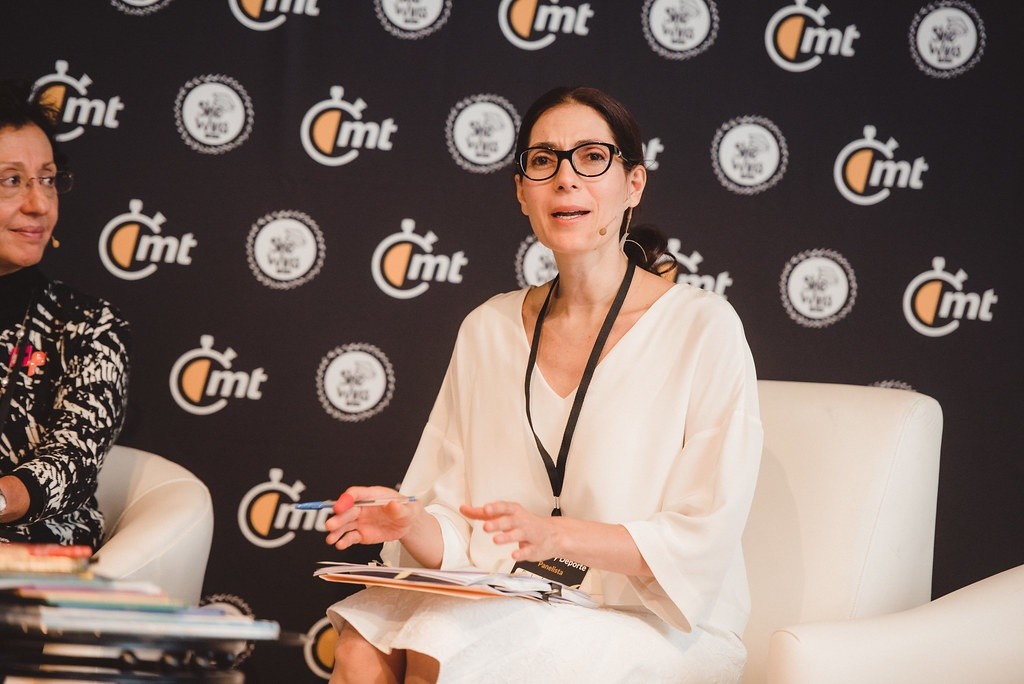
[0, 170, 74, 198]
[514, 142, 637, 181]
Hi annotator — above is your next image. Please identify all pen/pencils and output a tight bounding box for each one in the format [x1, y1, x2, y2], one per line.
[295, 496, 416, 509]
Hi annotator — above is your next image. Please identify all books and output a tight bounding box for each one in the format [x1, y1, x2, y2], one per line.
[0, 543, 280, 684]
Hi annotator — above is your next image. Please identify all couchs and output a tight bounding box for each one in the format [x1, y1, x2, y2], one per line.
[739, 380, 944, 682]
[769, 560, 1023, 683]
[4, 445, 213, 684]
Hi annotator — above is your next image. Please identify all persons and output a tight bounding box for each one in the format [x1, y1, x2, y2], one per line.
[326, 90, 765, 684]
[0, 104, 130, 561]
